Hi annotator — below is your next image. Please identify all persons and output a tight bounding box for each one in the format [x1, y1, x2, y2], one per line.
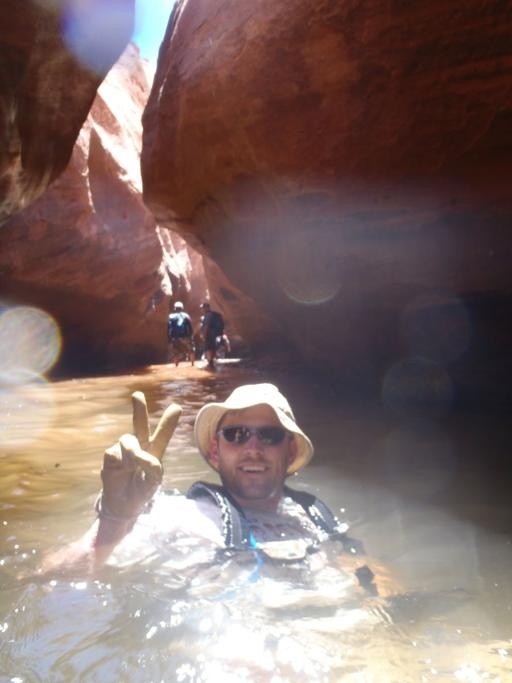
[41, 382, 368, 589]
[197, 303, 231, 368]
[167, 301, 195, 366]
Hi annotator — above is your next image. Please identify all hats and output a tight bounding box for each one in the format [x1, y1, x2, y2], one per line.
[194, 383, 314, 475]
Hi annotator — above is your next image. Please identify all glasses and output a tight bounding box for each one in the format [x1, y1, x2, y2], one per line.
[218, 425, 286, 446]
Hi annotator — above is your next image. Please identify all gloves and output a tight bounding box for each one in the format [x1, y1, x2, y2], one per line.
[94, 391, 183, 523]
